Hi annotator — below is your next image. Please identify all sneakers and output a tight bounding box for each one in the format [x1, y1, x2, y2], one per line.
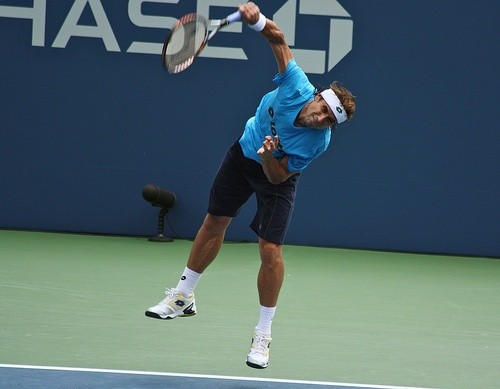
[246, 329, 273, 368]
[142, 290, 197, 320]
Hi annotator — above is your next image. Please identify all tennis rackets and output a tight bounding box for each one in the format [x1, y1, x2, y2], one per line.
[162, 10, 242, 74]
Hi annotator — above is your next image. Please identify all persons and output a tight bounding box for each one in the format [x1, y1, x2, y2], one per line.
[144, 1, 357, 368]
